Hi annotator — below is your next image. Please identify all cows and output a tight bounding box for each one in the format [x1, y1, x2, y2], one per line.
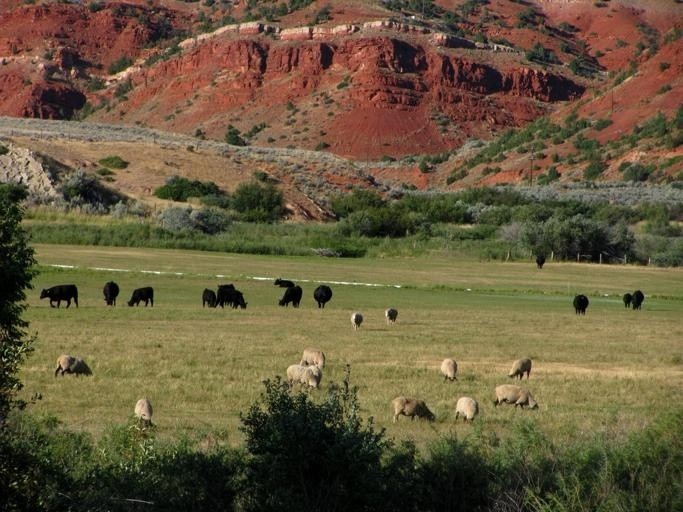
[314, 285, 332, 309]
[274, 277, 295, 288]
[623, 290, 644, 311]
[573, 294, 589, 316]
[103, 280, 119, 306]
[536, 255, 545, 269]
[40, 283, 78, 309]
[202, 283, 248, 310]
[279, 285, 302, 308]
[127, 286, 153, 307]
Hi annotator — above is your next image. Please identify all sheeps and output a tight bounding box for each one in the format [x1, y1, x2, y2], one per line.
[55, 355, 93, 378]
[391, 397, 436, 424]
[350, 311, 363, 331]
[385, 307, 398, 325]
[494, 384, 539, 411]
[287, 349, 325, 388]
[507, 358, 531, 381]
[455, 397, 478, 422]
[135, 398, 153, 421]
[441, 358, 458, 382]
